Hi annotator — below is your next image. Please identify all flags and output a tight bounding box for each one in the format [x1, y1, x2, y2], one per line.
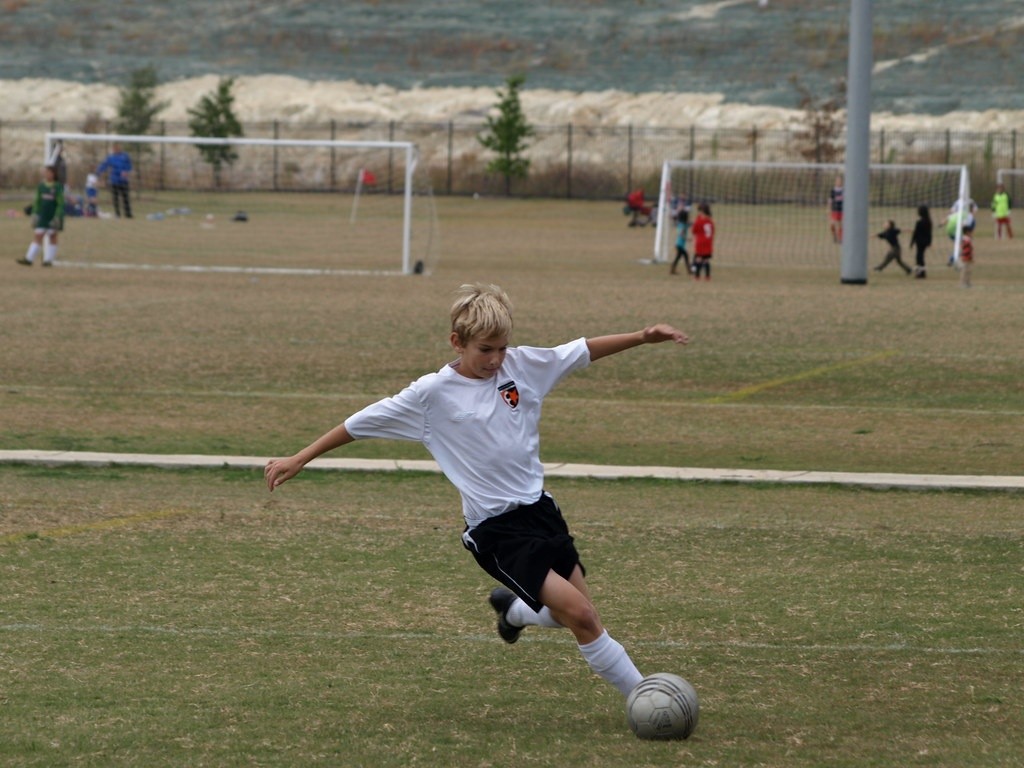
[361, 168, 378, 185]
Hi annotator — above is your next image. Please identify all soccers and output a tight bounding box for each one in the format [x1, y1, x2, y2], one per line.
[625, 671, 700, 741]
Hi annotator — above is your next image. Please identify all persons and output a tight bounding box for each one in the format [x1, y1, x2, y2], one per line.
[827, 174, 844, 244]
[937, 194, 978, 289]
[627, 182, 715, 282]
[85, 142, 134, 219]
[992, 183, 1014, 240]
[14, 138, 67, 267]
[868, 203, 933, 279]
[264, 285, 688, 698]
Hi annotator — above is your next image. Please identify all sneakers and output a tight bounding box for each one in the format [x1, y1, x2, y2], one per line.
[490, 589, 524, 645]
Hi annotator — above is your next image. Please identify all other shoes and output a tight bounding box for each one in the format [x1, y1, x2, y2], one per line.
[873, 266, 882, 272]
[668, 267, 679, 275]
[692, 274, 699, 281]
[41, 255, 52, 267]
[16, 257, 33, 266]
[704, 274, 711, 282]
[905, 269, 911, 276]
